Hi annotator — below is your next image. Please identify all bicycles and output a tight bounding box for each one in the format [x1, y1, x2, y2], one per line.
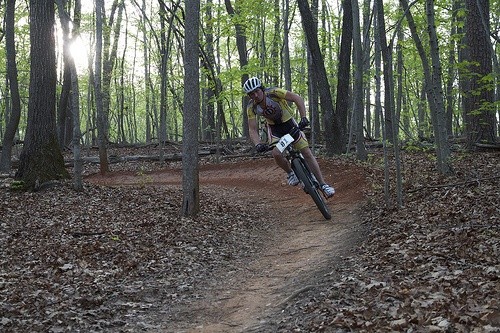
[262, 125, 332, 220]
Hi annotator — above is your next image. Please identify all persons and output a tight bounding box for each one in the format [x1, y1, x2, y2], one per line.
[242, 78, 336, 199]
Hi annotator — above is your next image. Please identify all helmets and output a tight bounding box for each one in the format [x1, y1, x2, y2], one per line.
[243, 77, 262, 94]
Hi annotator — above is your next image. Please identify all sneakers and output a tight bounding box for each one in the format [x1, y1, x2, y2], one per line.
[321, 184, 336, 200]
[287, 169, 299, 186]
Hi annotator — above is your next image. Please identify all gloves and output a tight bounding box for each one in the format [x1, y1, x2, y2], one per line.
[256, 144, 268, 153]
[298, 117, 309, 130]
